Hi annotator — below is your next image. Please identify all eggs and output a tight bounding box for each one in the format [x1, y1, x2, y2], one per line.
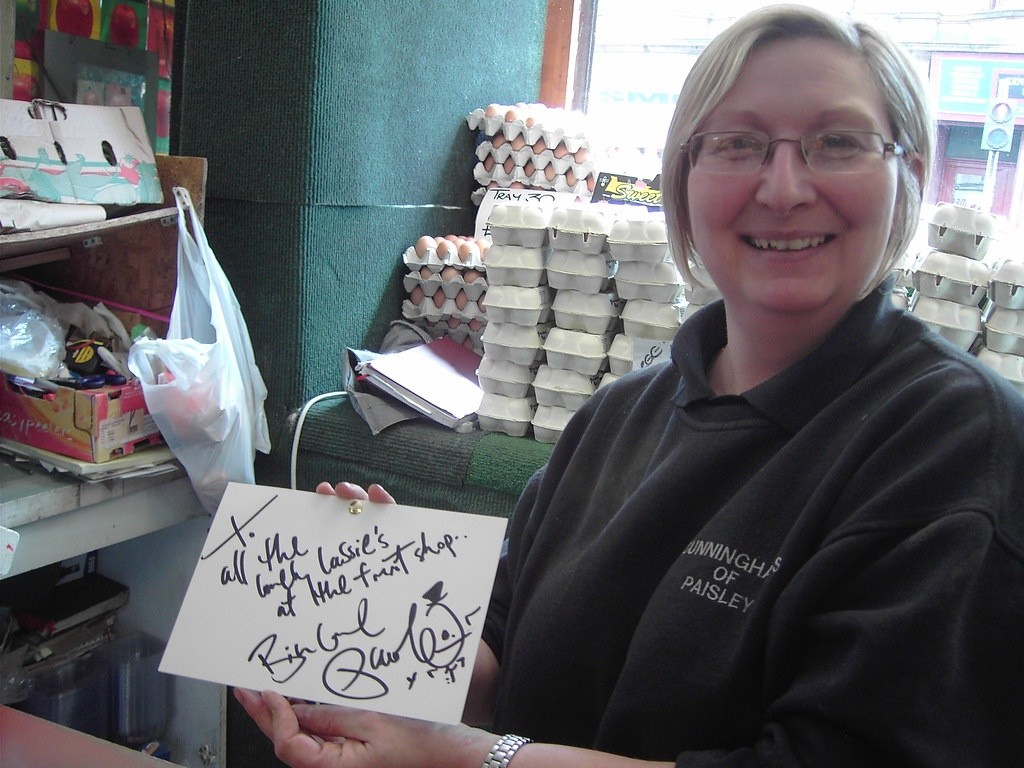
[481, 101, 596, 193]
[409, 234, 492, 350]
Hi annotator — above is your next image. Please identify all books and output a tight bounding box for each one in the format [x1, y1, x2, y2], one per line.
[14, 573, 129, 677]
[0, 437, 179, 483]
[358, 334, 484, 428]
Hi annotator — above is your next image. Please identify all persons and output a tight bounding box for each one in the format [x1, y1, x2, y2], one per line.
[232, 3, 1024, 768]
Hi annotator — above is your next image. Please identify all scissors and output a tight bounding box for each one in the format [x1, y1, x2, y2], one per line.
[50, 370, 125, 390]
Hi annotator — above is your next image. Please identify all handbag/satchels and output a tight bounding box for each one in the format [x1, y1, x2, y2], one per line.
[126, 187, 271, 516]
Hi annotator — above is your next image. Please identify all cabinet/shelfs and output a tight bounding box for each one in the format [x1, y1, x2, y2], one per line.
[0, 154, 226, 768]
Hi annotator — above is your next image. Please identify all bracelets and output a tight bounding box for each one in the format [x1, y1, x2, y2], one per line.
[482, 734, 534, 768]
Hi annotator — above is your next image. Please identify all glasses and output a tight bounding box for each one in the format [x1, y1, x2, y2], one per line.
[681, 130, 906, 175]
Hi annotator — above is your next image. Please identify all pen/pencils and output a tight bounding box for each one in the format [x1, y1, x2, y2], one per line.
[10, 458, 33, 476]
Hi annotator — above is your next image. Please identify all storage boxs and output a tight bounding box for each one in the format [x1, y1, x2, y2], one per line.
[0, 363, 165, 463]
[0, 99, 163, 212]
